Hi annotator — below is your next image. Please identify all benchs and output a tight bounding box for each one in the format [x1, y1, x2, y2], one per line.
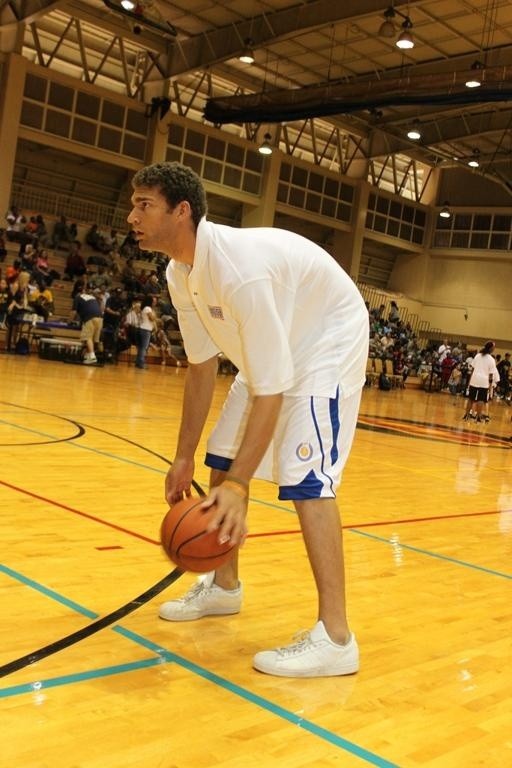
[0, 241, 186, 362]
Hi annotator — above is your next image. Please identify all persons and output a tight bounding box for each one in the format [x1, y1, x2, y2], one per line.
[362, 298, 511, 406]
[124, 160, 371, 679]
[462, 341, 495, 421]
[469, 355, 500, 419]
[1, 202, 240, 376]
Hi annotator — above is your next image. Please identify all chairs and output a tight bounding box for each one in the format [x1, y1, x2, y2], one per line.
[366, 358, 403, 389]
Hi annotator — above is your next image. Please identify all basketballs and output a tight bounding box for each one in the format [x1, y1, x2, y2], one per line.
[160, 497, 241, 573]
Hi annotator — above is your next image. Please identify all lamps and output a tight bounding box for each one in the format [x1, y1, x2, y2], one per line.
[238, 0, 481, 155]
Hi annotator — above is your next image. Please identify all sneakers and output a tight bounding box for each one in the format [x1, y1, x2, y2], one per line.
[252, 619, 359, 679]
[462, 415, 490, 424]
[82, 358, 98, 364]
[158, 570, 243, 622]
